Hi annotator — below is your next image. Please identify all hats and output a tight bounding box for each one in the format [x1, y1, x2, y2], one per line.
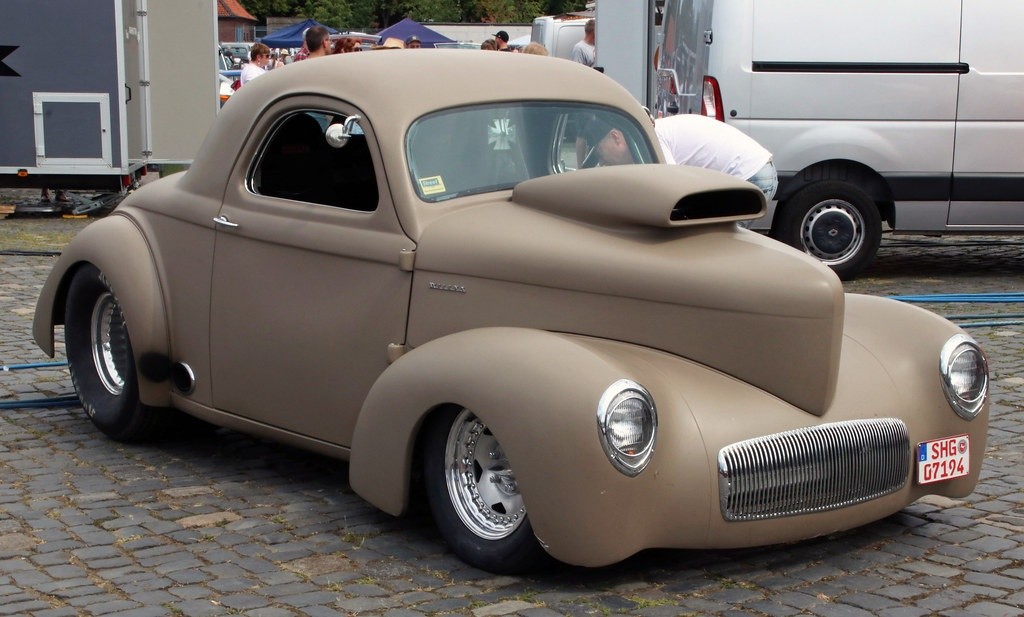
[405, 36, 421, 45]
[580, 120, 614, 169]
[493, 30, 509, 43]
[371, 37, 405, 49]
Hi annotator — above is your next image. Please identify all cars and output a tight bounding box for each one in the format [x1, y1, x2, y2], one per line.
[31, 50, 991, 571]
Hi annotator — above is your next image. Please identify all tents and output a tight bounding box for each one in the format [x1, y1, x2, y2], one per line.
[254, 19, 338, 55]
[373, 17, 456, 47]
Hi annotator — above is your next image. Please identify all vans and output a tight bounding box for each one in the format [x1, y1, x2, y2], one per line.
[220, 43, 255, 60]
[531, 0, 596, 61]
[218, 73, 234, 103]
[596, 0, 1023, 281]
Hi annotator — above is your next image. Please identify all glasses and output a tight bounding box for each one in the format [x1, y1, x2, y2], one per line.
[264, 55, 269, 58]
[411, 44, 419, 48]
[355, 48, 362, 51]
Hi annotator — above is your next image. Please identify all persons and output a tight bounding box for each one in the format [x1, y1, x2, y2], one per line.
[481, 30, 554, 160]
[255, 25, 363, 70]
[240, 43, 270, 87]
[582, 106, 778, 230]
[406, 33, 422, 49]
[571, 18, 596, 170]
